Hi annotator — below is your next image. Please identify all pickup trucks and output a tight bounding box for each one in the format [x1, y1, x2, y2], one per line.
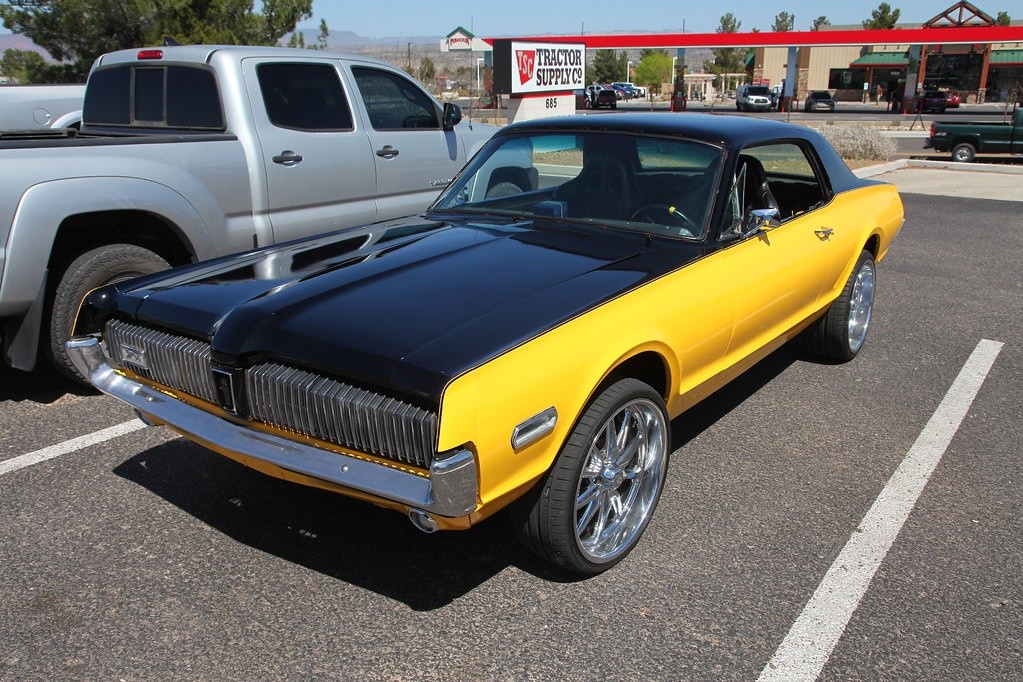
[0, 43, 541, 389]
[922, 107, 1023, 164]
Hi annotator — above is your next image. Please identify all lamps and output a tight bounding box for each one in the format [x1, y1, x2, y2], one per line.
[937, 46, 944, 59]
[968, 45, 977, 57]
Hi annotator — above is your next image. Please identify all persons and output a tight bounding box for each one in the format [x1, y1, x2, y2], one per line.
[876, 85, 883, 105]
[591, 89, 595, 101]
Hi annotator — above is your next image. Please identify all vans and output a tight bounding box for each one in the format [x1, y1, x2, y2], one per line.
[735, 85, 773, 112]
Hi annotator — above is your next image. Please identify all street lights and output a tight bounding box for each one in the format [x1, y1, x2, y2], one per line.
[477, 57, 484, 88]
[627, 61, 633, 83]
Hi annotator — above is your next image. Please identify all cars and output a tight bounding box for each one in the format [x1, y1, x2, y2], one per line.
[584, 81, 647, 108]
[805, 89, 837, 112]
[64, 109, 905, 577]
[920, 90, 961, 113]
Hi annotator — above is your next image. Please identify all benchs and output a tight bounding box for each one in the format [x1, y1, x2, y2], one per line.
[640, 174, 823, 222]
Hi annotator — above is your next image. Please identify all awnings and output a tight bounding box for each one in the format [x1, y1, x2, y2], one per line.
[745, 54, 755, 70]
[849, 52, 909, 68]
[988, 50, 1023, 68]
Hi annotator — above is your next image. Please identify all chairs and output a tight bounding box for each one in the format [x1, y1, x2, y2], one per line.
[552, 136, 658, 222]
[172, 69, 223, 128]
[261, 76, 294, 127]
[681, 154, 780, 237]
